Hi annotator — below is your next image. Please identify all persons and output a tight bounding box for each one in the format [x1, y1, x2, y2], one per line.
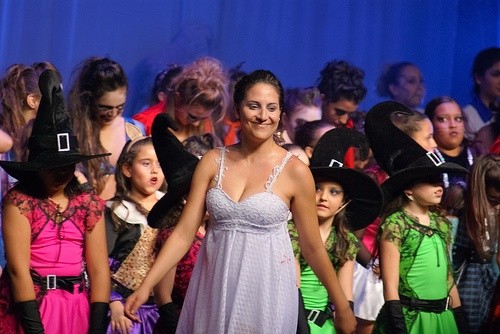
[124, 69, 358, 334]
[0, 55, 249, 334]
[275, 47, 499, 334]
[0, 68, 114, 334]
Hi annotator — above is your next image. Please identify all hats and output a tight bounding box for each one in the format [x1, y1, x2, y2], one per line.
[305, 127, 383, 231]
[363, 101, 468, 208]
[0, 68, 111, 180]
[147, 111, 200, 229]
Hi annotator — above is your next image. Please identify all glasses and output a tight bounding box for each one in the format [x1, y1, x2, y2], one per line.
[89, 102, 127, 111]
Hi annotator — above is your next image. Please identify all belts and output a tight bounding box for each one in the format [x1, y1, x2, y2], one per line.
[304, 307, 335, 327]
[32, 274, 84, 294]
[400, 295, 450, 314]
